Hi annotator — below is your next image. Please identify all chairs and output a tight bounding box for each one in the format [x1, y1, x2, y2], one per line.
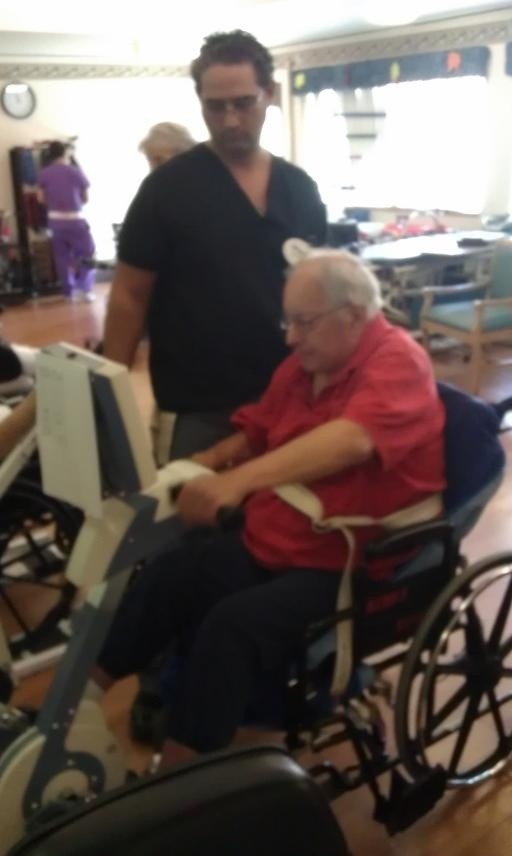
[419, 238, 511, 399]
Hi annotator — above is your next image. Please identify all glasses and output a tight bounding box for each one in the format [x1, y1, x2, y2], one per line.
[279, 299, 352, 334]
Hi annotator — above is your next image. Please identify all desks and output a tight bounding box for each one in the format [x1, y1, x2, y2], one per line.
[359, 230, 511, 358]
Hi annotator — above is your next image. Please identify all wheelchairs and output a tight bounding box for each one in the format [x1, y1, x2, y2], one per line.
[124, 380, 512, 837]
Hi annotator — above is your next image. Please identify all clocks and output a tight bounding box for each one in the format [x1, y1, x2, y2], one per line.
[1, 81, 37, 119]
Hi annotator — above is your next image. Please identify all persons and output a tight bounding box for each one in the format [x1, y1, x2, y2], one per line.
[0, 335, 106, 465]
[90, 245, 449, 777]
[32, 137, 100, 304]
[135, 119, 200, 173]
[102, 24, 332, 471]
